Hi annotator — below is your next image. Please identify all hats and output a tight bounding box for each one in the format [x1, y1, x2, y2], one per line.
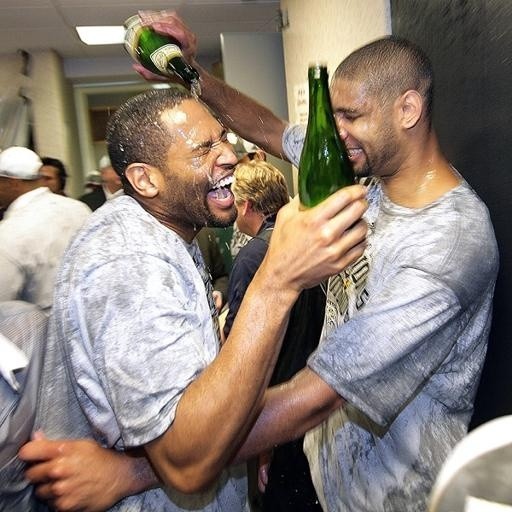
[0, 147, 44, 181]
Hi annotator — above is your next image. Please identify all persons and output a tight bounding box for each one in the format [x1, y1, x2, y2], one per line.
[0, 145, 328, 511]
[28, 87, 372, 511]
[13, 4, 502, 512]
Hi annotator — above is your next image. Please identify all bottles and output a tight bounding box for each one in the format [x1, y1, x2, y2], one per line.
[296, 64, 360, 208]
[114, 14, 199, 86]
